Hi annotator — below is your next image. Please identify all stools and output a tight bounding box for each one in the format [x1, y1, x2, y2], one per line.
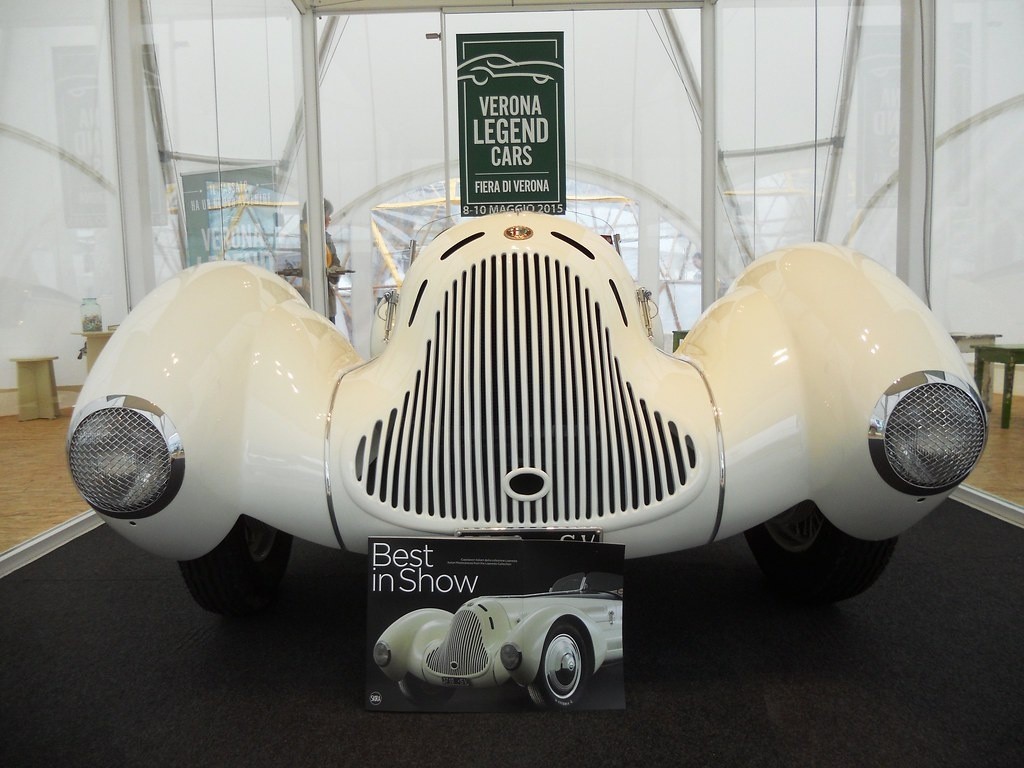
[9, 356, 62, 422]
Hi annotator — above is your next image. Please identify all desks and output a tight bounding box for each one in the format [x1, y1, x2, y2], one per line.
[949, 333, 1003, 412]
[969, 343, 1024, 429]
[71, 330, 115, 374]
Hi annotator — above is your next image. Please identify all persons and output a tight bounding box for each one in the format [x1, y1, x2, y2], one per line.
[693, 252, 702, 282]
[300, 198, 346, 327]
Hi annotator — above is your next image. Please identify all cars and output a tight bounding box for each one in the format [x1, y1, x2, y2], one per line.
[375, 571, 622, 708]
[65, 207, 990, 605]
